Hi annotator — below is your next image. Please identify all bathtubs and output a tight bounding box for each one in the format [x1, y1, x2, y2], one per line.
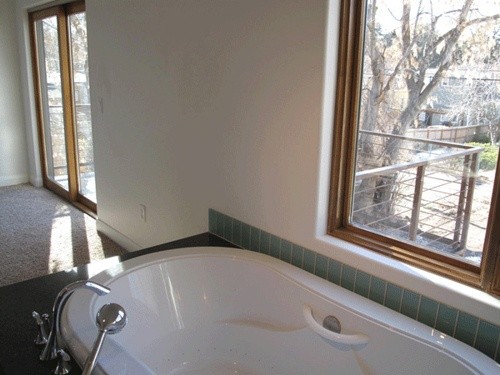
[58, 246, 500, 375]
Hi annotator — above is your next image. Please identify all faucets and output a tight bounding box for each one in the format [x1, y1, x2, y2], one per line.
[38, 279, 112, 363]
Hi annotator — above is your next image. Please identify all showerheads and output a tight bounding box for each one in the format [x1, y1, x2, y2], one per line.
[81, 301, 127, 374]
